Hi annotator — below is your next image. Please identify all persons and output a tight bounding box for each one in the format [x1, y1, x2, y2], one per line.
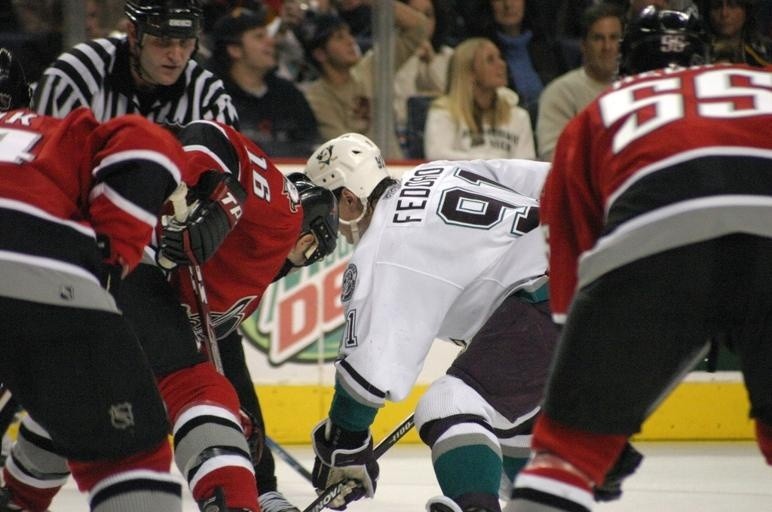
[1, 1, 772, 159]
[500, 0, 772, 512]
[304, 133, 645, 512]
[4, 3, 337, 512]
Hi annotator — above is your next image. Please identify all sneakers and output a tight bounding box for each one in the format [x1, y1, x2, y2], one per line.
[258, 490, 301, 511]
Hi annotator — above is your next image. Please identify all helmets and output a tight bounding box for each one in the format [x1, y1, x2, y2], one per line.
[618, 5, 712, 73]
[287, 132, 389, 265]
[124, 0, 203, 39]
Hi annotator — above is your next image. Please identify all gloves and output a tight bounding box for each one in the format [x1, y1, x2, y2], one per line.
[160, 168, 253, 267]
[310, 419, 378, 510]
